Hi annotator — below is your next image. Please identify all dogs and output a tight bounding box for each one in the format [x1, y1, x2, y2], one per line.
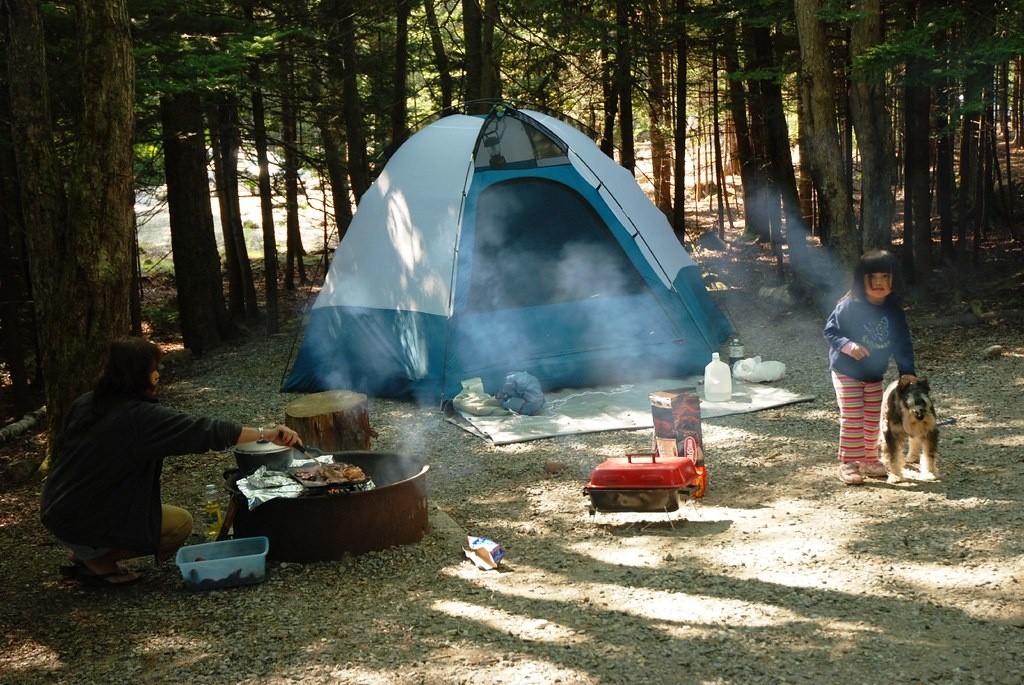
[876, 376, 941, 484]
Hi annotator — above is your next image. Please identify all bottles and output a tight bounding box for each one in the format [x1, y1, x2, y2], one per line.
[704, 352, 732, 401]
[204, 484, 223, 535]
[729, 338, 745, 371]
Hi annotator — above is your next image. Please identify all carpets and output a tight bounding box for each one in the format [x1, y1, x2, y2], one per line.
[442, 376, 816, 446]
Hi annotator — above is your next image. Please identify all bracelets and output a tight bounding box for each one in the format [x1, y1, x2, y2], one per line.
[257, 427, 264, 442]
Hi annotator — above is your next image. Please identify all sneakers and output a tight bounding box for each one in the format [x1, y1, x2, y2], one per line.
[859, 462, 887, 477]
[837, 463, 863, 484]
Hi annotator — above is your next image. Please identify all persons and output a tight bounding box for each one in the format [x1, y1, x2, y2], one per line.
[40, 338, 302, 589]
[822, 250, 920, 484]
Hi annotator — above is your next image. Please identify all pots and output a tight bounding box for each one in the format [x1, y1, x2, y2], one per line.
[234, 439, 292, 474]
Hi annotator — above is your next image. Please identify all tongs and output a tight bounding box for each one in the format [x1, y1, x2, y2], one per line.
[279, 431, 336, 477]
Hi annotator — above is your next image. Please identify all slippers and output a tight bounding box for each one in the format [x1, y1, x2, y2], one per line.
[70, 554, 140, 587]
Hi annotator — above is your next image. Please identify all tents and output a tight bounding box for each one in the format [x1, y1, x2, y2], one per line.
[279, 96, 741, 410]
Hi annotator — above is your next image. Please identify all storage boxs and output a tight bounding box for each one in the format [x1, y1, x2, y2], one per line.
[175, 536, 269, 593]
